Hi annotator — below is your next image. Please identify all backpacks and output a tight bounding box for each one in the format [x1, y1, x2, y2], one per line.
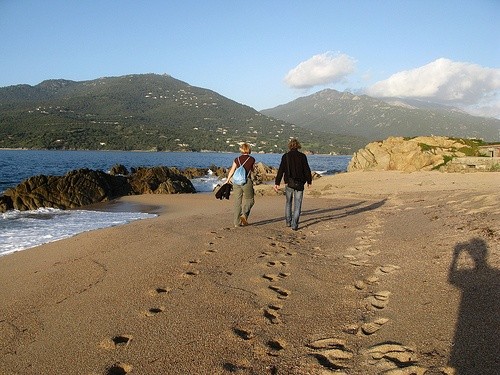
[232, 156, 251, 185]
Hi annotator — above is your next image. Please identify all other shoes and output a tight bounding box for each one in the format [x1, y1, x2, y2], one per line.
[235, 224, 240, 227]
[240, 214, 249, 226]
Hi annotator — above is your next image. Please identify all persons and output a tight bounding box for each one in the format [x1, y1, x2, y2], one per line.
[273, 138, 313, 232]
[224, 145, 257, 229]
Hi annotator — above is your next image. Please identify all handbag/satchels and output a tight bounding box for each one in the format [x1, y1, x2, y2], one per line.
[289, 176, 304, 191]
